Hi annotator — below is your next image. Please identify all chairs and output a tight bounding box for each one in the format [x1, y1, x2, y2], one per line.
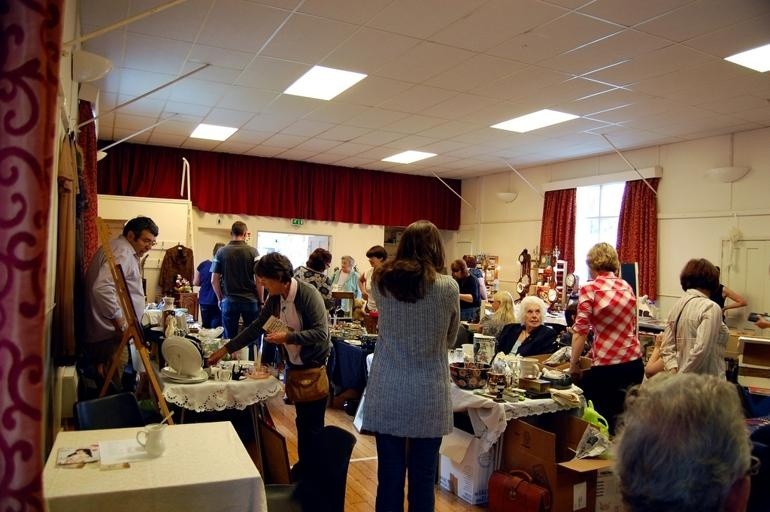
[263, 423, 355, 512]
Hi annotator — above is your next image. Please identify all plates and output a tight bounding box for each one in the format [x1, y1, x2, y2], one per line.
[162, 335, 202, 375]
[161, 366, 208, 383]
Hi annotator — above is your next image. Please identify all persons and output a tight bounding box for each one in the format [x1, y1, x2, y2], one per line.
[450, 260, 480, 323]
[494, 296, 557, 361]
[552, 303, 593, 361]
[645, 330, 667, 377]
[83, 217, 159, 393]
[709, 266, 747, 324]
[561, 242, 644, 438]
[203, 252, 336, 483]
[359, 245, 388, 316]
[332, 256, 362, 304]
[659, 258, 731, 386]
[192, 243, 226, 341]
[362, 219, 461, 512]
[752, 310, 765, 327]
[209, 222, 265, 360]
[462, 255, 487, 303]
[467, 289, 516, 348]
[292, 248, 336, 315]
[611, 371, 752, 509]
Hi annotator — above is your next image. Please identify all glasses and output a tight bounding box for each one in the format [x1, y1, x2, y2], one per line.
[138, 238, 157, 247]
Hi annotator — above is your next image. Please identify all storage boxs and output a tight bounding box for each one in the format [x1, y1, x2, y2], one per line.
[503, 417, 616, 511]
[438, 428, 494, 506]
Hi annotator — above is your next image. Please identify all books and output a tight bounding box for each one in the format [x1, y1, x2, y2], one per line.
[263, 314, 294, 335]
[54, 443, 100, 466]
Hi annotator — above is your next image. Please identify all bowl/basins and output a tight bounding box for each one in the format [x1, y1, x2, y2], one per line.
[449, 362, 492, 390]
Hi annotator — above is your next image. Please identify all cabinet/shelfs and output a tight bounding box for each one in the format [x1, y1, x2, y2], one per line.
[520, 259, 569, 313]
[476, 254, 499, 303]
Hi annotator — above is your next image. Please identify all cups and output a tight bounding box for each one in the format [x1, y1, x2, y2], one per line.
[520, 358, 539, 378]
[136, 424, 168, 457]
[164, 298, 173, 309]
